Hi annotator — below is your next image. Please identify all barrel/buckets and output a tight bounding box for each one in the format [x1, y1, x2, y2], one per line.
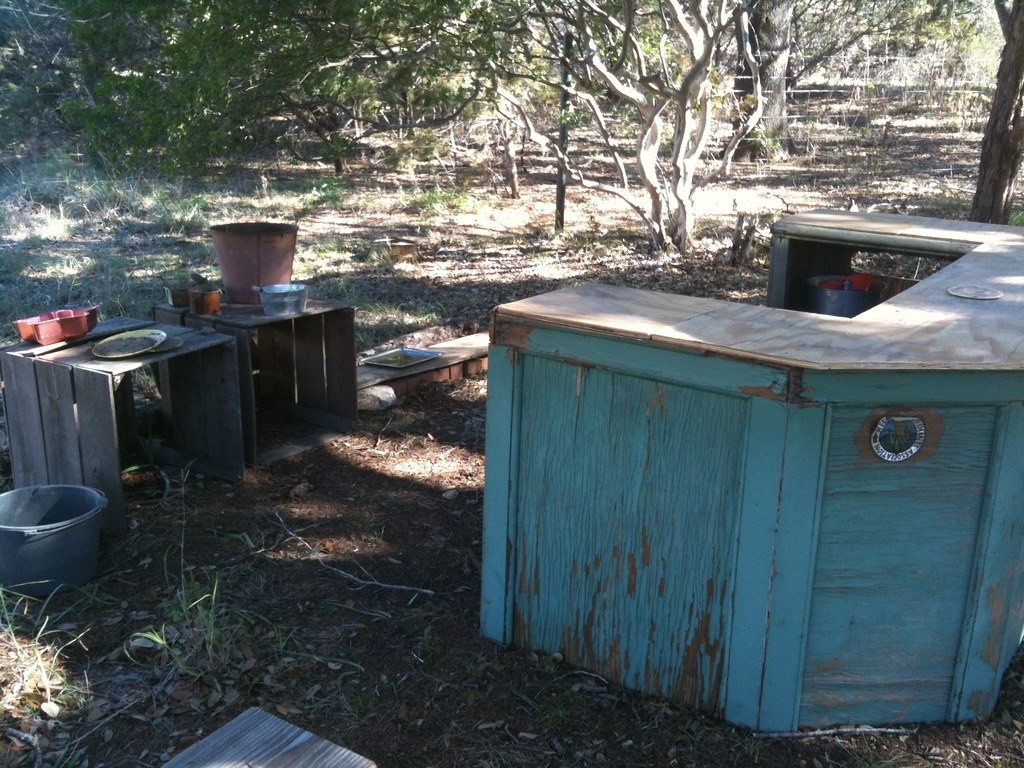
[259, 283, 308, 316]
[809, 275, 888, 317]
[0, 483, 108, 599]
[211, 222, 300, 304]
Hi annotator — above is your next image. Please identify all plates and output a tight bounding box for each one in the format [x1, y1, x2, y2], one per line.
[91, 328, 168, 358]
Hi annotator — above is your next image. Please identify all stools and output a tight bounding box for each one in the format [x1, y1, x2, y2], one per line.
[160, 706, 378, 768]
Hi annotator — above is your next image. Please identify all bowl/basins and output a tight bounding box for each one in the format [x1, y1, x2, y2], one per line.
[15, 309, 90, 346]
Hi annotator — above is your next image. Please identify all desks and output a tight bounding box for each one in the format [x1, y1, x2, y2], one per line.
[479, 209, 1024, 734]
